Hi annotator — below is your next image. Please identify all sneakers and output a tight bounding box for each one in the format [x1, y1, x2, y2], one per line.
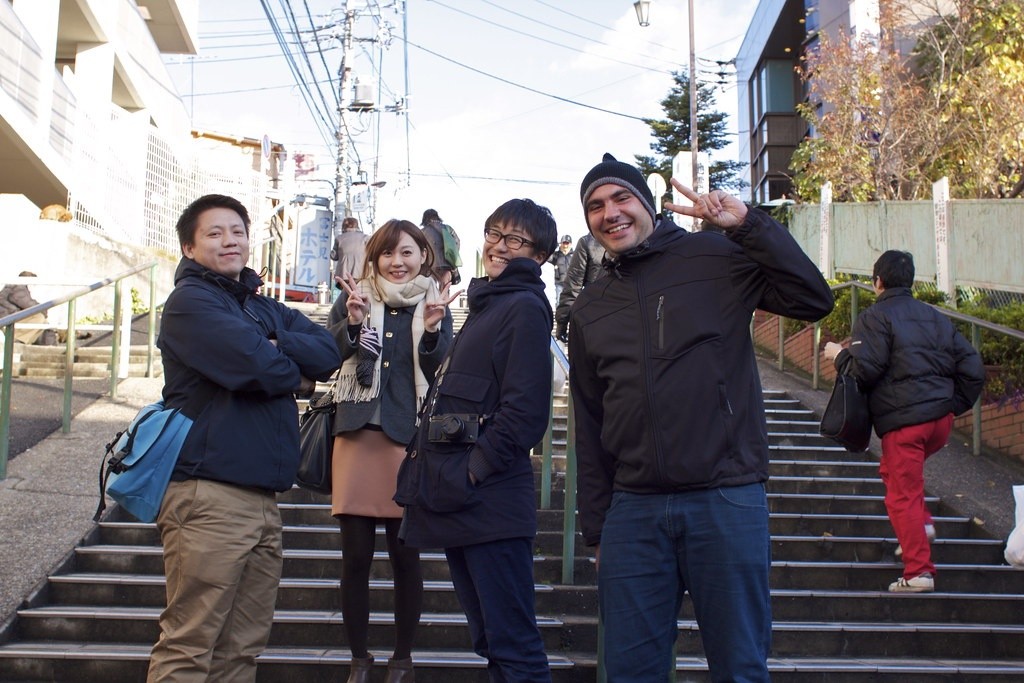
[888, 571, 934, 593]
[895, 523, 936, 559]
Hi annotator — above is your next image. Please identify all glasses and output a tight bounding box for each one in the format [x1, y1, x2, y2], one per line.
[484, 227, 536, 250]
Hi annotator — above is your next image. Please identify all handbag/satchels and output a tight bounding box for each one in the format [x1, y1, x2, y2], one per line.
[450, 266, 461, 285]
[294, 394, 336, 496]
[105, 400, 195, 523]
[819, 358, 872, 454]
[1002, 484, 1024, 570]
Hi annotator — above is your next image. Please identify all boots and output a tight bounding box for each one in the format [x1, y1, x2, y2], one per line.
[347, 652, 374, 683]
[384, 656, 415, 683]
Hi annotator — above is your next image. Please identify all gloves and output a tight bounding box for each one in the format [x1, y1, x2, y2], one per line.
[556, 323, 568, 342]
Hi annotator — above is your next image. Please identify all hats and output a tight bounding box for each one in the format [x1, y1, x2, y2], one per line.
[580, 153, 657, 239]
[19, 271, 37, 277]
[419, 209, 443, 225]
[561, 235, 572, 243]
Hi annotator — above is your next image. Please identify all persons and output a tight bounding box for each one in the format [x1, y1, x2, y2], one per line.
[555, 232, 612, 343]
[392, 196, 558, 683]
[146, 194, 342, 683]
[419, 209, 460, 296]
[568, 162, 835, 683]
[824, 248, 986, 593]
[327, 219, 464, 683]
[0, 271, 47, 344]
[330, 216, 371, 291]
[546, 235, 574, 308]
